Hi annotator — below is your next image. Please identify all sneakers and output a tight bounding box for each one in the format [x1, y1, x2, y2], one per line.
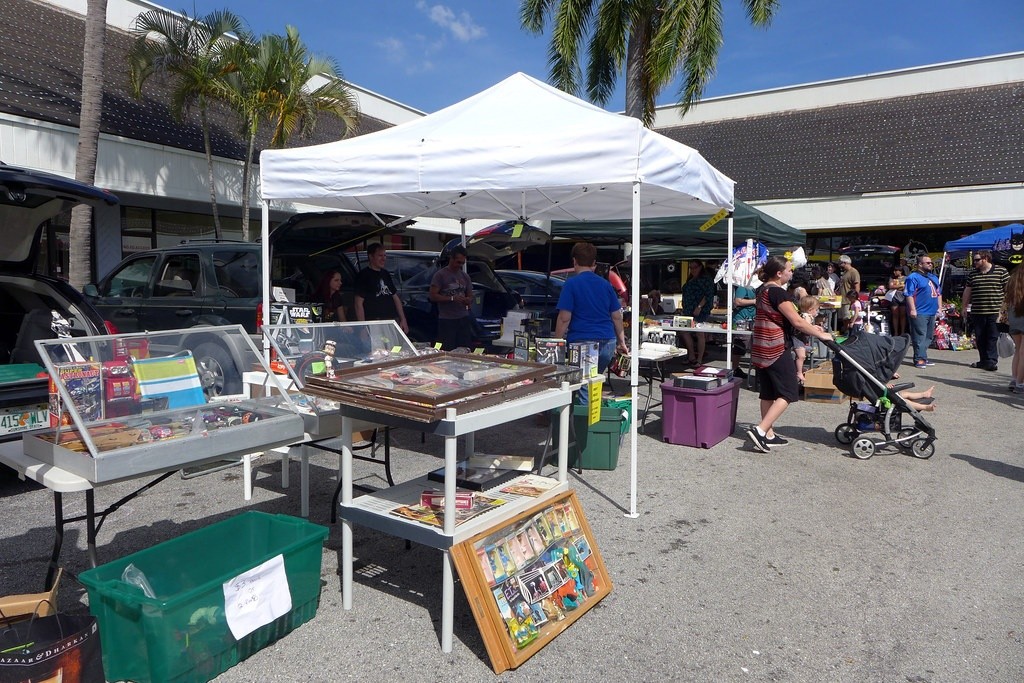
[746, 426, 770, 452]
[766, 434, 788, 446]
[914, 360, 935, 368]
[732, 366, 748, 379]
[1008, 380, 1024, 393]
[997, 332, 1015, 358]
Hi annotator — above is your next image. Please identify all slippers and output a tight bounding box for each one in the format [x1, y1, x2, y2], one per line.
[680, 358, 702, 369]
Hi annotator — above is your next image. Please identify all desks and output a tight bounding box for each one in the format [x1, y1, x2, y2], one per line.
[0, 433, 329, 593]
[624, 348, 687, 435]
[241, 369, 373, 516]
[492, 339, 514, 347]
[662, 326, 754, 388]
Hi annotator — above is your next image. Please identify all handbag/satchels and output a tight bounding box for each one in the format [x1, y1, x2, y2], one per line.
[885, 289, 897, 302]
[0, 600, 105, 683]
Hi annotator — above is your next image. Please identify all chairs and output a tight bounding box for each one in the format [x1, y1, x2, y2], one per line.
[168, 270, 197, 296]
[127, 349, 243, 479]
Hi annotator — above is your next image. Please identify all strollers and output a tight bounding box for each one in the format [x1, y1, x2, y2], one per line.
[818, 334, 939, 459]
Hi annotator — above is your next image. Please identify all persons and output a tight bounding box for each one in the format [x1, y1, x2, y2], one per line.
[902, 254, 943, 369]
[731, 255, 835, 453]
[429, 245, 475, 353]
[885, 373, 936, 411]
[960, 250, 1011, 371]
[304, 270, 348, 323]
[1005, 259, 1024, 394]
[554, 242, 629, 406]
[888, 264, 908, 337]
[786, 254, 863, 338]
[642, 290, 664, 315]
[675, 259, 715, 368]
[352, 242, 410, 335]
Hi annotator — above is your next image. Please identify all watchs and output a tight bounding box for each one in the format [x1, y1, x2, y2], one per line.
[697, 305, 701, 308]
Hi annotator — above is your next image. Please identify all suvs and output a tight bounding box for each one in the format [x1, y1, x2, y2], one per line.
[840, 245, 904, 324]
[280, 219, 555, 351]
[0, 163, 140, 442]
[81, 210, 419, 402]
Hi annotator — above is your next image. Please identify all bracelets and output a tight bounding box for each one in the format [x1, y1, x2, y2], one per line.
[451, 296, 454, 301]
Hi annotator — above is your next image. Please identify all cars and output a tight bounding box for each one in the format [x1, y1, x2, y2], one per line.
[494, 269, 566, 331]
[552, 259, 629, 306]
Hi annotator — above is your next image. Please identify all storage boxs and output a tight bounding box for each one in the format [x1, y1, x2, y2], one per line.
[802, 360, 867, 404]
[76, 509, 329, 683]
[23, 323, 305, 483]
[449, 489, 613, 675]
[672, 316, 695, 328]
[269, 301, 323, 374]
[550, 404, 622, 471]
[260, 319, 419, 436]
[659, 375, 743, 449]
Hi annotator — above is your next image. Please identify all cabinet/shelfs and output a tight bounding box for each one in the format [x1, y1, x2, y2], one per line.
[299, 352, 558, 423]
[339, 381, 571, 650]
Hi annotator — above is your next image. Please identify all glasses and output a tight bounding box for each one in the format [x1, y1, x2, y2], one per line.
[973, 259, 981, 262]
[689, 266, 699, 269]
[926, 262, 933, 264]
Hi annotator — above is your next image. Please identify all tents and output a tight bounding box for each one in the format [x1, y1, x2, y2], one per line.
[258, 71, 806, 519]
[939, 223, 1024, 285]
